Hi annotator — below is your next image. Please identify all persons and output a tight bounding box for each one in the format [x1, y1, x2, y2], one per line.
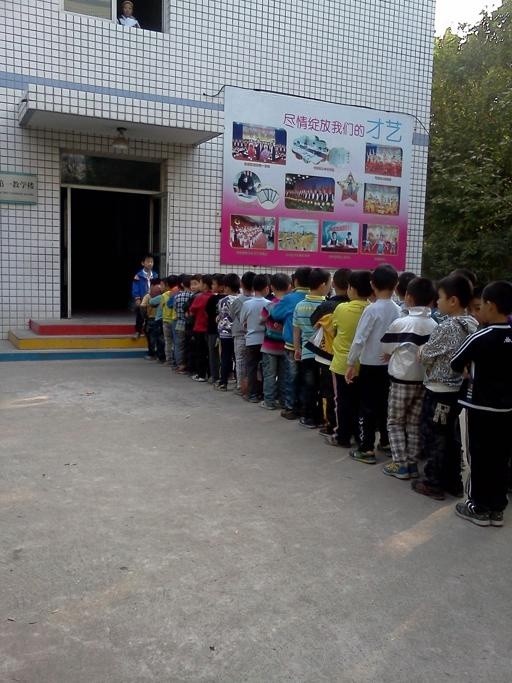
[116, 1, 142, 28]
[230, 137, 402, 255]
[143, 262, 510, 527]
[132, 253, 159, 340]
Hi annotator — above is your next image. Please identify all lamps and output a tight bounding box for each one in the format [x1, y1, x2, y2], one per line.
[112, 127, 128, 149]
